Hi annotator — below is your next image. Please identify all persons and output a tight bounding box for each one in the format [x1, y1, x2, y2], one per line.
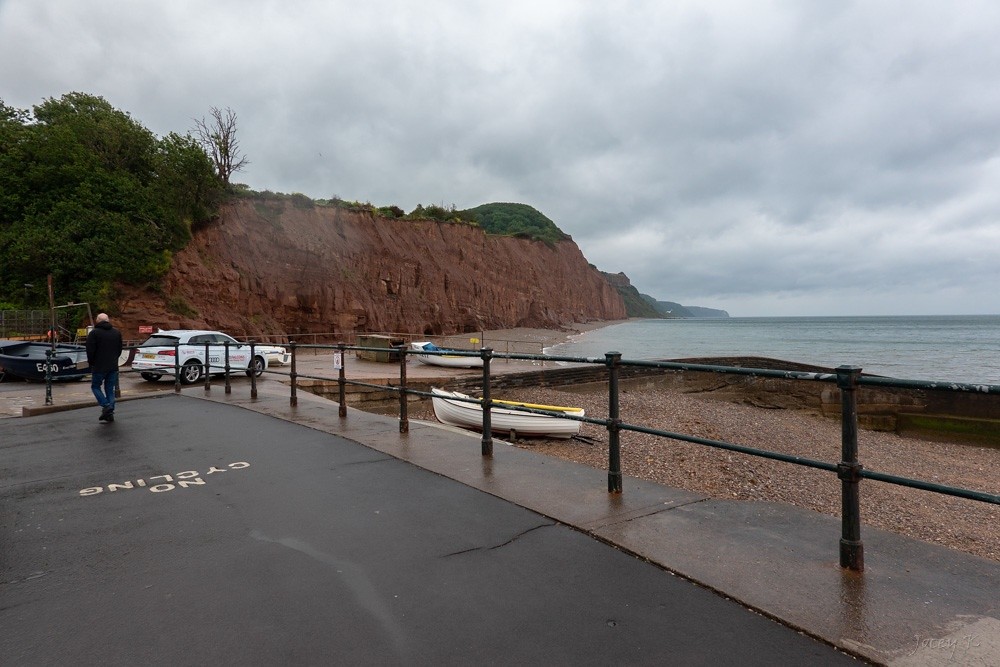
[85, 312, 123, 422]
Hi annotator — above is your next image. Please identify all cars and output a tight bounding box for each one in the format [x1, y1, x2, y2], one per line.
[132, 330, 268, 385]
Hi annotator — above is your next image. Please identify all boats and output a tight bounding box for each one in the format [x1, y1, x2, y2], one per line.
[0, 276, 91, 381]
[410, 341, 495, 368]
[256, 346, 292, 367]
[430, 385, 586, 439]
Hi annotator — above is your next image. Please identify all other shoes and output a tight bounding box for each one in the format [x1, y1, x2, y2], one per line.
[102, 405, 114, 415]
[99, 414, 114, 422]
